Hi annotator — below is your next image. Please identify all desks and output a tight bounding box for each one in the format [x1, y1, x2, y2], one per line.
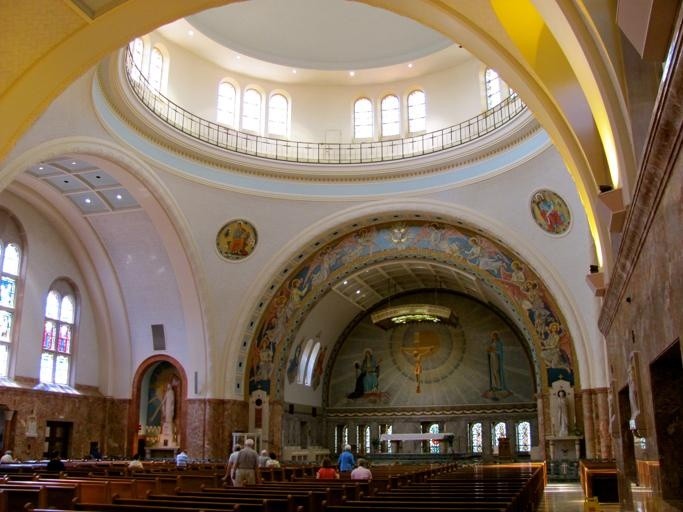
[579, 458, 620, 508]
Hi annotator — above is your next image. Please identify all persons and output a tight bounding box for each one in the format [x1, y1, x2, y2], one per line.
[556, 385, 570, 436]
[361, 348, 381, 392]
[540, 322, 571, 374]
[337, 445, 356, 472]
[486, 332, 505, 392]
[353, 361, 366, 396]
[535, 191, 566, 232]
[228, 222, 251, 258]
[46, 449, 66, 472]
[175, 447, 188, 471]
[402, 347, 434, 394]
[219, 435, 280, 489]
[127, 453, 143, 469]
[249, 336, 273, 383]
[263, 228, 373, 342]
[92, 446, 102, 459]
[160, 382, 174, 423]
[316, 457, 340, 479]
[350, 458, 372, 484]
[0, 449, 16, 462]
[427, 220, 551, 346]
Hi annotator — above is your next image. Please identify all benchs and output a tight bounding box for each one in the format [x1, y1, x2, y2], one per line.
[0, 458, 546, 511]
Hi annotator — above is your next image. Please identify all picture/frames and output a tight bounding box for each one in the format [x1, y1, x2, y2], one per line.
[214, 217, 260, 264]
[526, 187, 575, 238]
